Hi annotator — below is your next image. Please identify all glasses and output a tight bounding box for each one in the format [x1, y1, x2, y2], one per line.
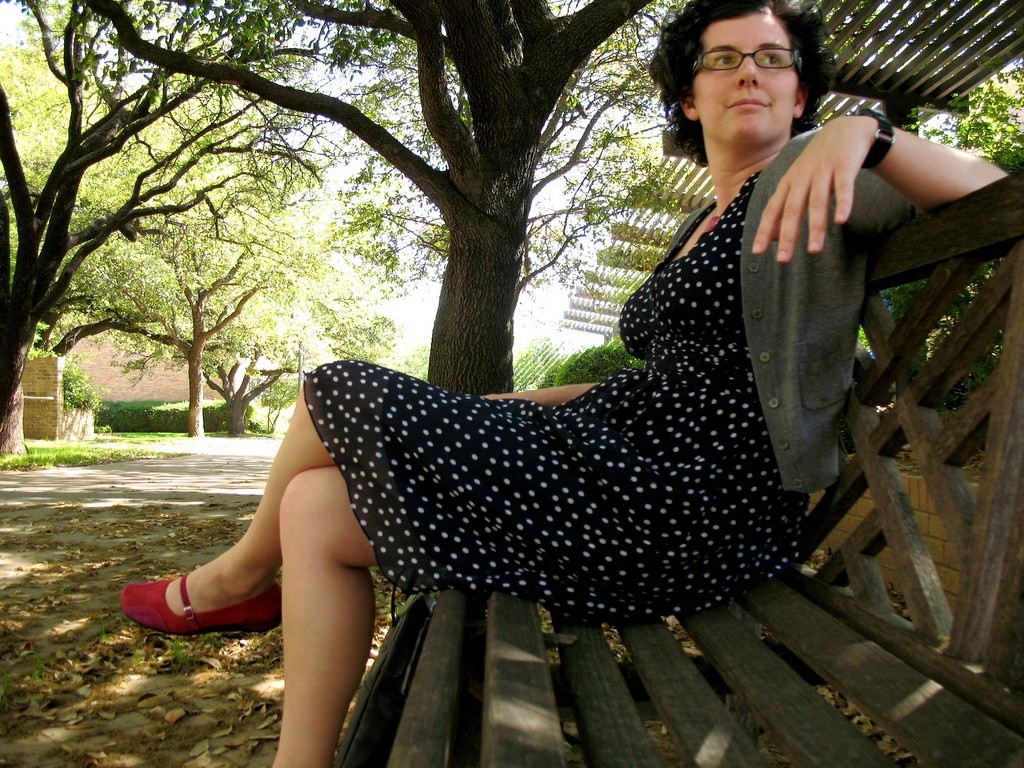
[692, 48, 802, 77]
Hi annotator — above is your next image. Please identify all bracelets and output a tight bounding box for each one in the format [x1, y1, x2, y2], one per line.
[858, 108, 896, 169]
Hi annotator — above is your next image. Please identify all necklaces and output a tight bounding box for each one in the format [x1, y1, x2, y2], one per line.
[705, 216, 720, 231]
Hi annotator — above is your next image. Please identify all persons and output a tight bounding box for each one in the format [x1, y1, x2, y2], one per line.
[121, 0, 1024, 768]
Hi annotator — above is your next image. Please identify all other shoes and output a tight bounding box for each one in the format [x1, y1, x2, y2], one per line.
[120, 574, 284, 635]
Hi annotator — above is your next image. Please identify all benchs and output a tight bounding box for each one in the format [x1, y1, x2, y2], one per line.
[333, 164, 1024, 768]
